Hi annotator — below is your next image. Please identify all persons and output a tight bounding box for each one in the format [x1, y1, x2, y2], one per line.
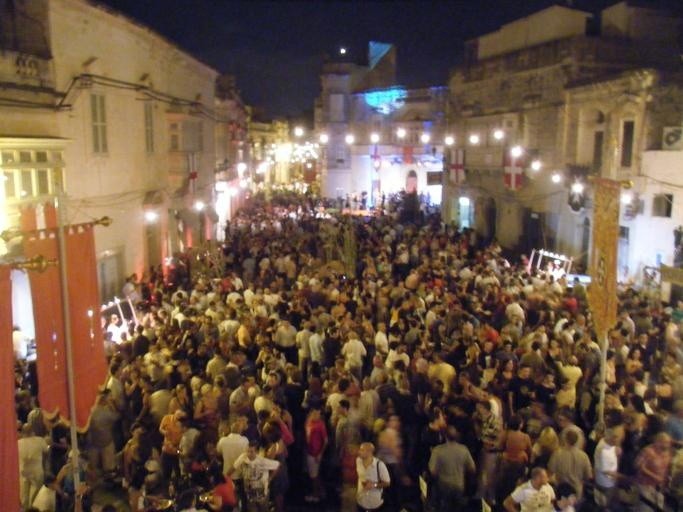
[10, 182, 683, 512]
